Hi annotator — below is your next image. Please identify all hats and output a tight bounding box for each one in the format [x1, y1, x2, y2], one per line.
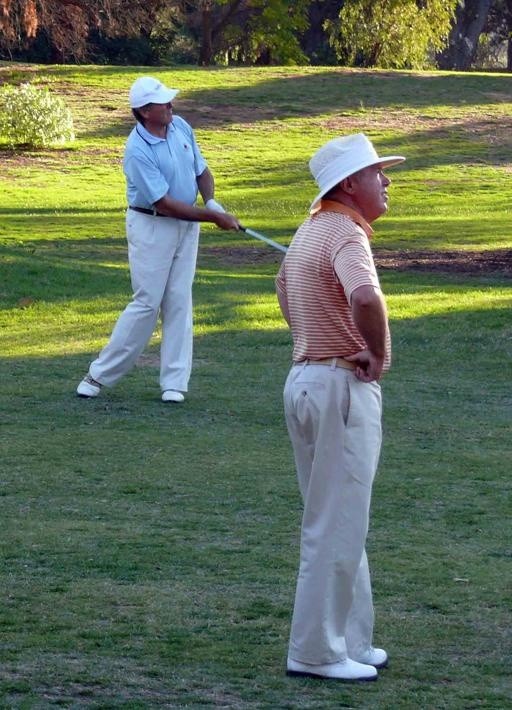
[309, 133, 406, 214]
[129, 77, 180, 108]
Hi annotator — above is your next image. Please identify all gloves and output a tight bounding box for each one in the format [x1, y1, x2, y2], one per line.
[205, 200, 224, 213]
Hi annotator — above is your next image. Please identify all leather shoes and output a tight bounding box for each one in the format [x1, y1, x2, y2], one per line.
[363, 649, 388, 668]
[77, 371, 100, 397]
[287, 655, 378, 683]
[162, 390, 184, 402]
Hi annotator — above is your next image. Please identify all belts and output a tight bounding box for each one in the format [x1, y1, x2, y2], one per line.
[294, 358, 359, 369]
[130, 206, 168, 217]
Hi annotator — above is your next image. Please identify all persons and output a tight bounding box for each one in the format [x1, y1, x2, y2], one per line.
[268, 134, 408, 688]
[72, 74, 245, 406]
